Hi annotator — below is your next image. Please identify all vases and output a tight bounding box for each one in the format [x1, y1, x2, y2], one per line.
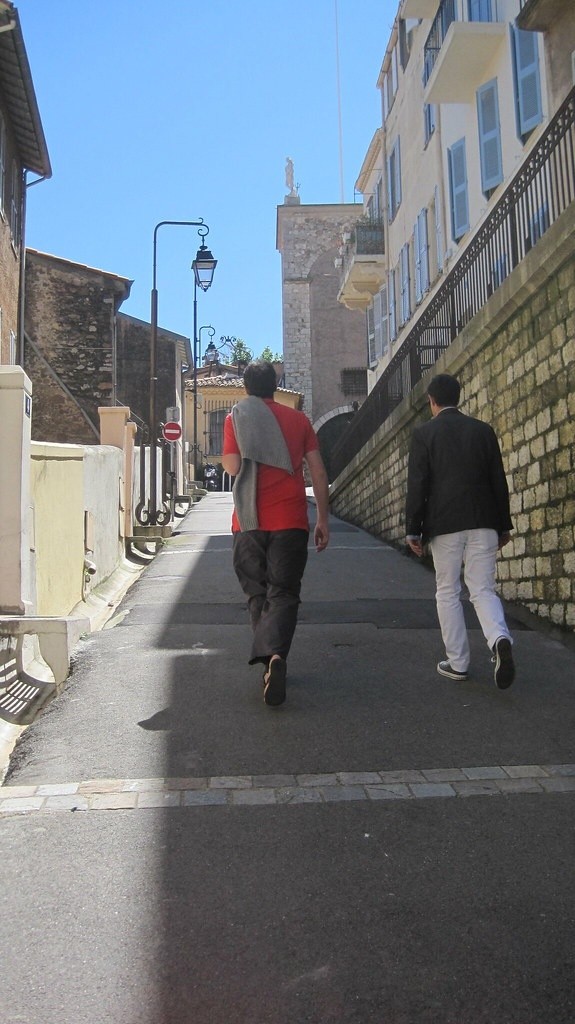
[335, 259, 342, 269]
[344, 232, 351, 241]
[338, 245, 346, 255]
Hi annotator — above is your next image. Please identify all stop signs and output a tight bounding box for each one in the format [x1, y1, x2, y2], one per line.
[162, 423, 182, 442]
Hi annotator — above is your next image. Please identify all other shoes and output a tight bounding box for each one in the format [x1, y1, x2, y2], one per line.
[263, 655, 288, 706]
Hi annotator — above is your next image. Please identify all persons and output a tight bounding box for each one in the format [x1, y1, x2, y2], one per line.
[220, 359, 330, 707]
[404, 374, 514, 689]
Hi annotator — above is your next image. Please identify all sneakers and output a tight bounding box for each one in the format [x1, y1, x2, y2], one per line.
[437, 659, 468, 680]
[491, 635, 516, 689]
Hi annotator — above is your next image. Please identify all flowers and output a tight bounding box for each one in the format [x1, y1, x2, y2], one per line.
[341, 222, 354, 233]
[333, 252, 344, 261]
[336, 237, 346, 246]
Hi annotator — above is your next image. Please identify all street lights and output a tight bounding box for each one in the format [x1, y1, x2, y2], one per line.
[135, 218, 218, 525]
[189, 324, 217, 481]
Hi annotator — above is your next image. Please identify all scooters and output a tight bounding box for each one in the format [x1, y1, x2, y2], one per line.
[203, 455, 218, 491]
